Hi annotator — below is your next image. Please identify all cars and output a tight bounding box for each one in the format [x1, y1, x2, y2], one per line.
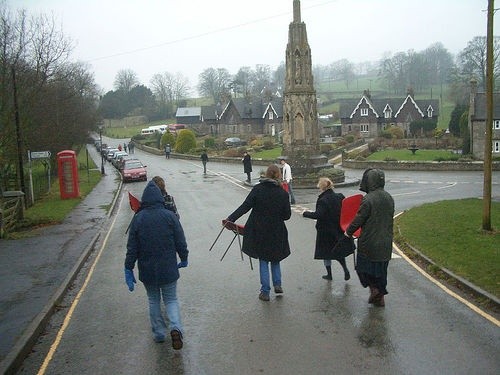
[224, 137, 245, 146]
[94, 138, 148, 182]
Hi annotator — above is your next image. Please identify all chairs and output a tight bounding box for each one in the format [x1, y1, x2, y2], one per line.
[328, 194, 366, 269]
[209, 219, 254, 271]
[125, 192, 141, 233]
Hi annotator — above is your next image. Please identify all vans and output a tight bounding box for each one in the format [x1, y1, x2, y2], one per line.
[141, 125, 168, 136]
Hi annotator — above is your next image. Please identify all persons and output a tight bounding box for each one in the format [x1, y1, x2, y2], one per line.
[345, 167, 395, 307]
[242, 152, 253, 183]
[200, 150, 209, 174]
[279, 157, 294, 204]
[125, 181, 189, 350]
[300, 178, 356, 280]
[153, 176, 180, 219]
[164, 144, 171, 159]
[118, 143, 127, 152]
[128, 139, 136, 154]
[226, 164, 291, 301]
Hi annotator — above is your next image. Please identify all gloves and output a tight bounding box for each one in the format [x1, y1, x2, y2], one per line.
[178, 260, 188, 268]
[125, 269, 137, 291]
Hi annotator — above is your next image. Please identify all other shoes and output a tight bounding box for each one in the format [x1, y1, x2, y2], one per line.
[275, 287, 283, 293]
[374, 296, 385, 307]
[370, 288, 384, 303]
[259, 292, 269, 300]
[171, 331, 183, 349]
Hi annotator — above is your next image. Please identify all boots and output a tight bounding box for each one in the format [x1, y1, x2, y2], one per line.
[342, 264, 350, 280]
[322, 266, 332, 280]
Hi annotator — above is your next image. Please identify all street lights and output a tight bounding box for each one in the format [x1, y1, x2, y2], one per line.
[98, 125, 104, 174]
